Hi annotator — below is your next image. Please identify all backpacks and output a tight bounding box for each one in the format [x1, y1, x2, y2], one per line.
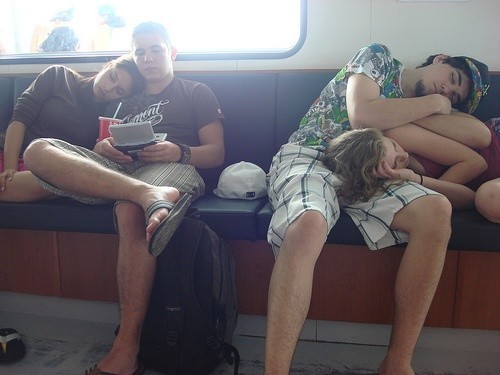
[115, 208, 240, 375]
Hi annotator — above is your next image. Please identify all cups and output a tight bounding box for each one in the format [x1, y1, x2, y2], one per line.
[99, 117, 123, 141]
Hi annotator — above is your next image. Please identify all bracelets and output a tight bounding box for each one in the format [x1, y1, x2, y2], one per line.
[419, 174, 424, 185]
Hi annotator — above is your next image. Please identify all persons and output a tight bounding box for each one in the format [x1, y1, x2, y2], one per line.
[264, 43, 491, 375]
[93, 5, 127, 50]
[30, 9, 75, 53]
[0, 55, 145, 201]
[23, 21, 224, 375]
[324, 110, 500, 223]
[39, 27, 81, 54]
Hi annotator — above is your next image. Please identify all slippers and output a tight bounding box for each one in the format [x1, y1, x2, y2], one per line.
[144, 192, 192, 256]
[82, 360, 144, 375]
[0, 328, 25, 365]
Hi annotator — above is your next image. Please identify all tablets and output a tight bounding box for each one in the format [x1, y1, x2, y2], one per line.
[109, 121, 168, 159]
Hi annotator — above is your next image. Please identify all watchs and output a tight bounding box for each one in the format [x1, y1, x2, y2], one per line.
[177, 143, 192, 164]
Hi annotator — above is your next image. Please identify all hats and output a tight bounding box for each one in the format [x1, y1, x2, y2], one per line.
[212, 161, 267, 200]
[456, 55, 489, 114]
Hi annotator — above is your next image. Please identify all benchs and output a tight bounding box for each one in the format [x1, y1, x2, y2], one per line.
[0, 68, 500, 353]
